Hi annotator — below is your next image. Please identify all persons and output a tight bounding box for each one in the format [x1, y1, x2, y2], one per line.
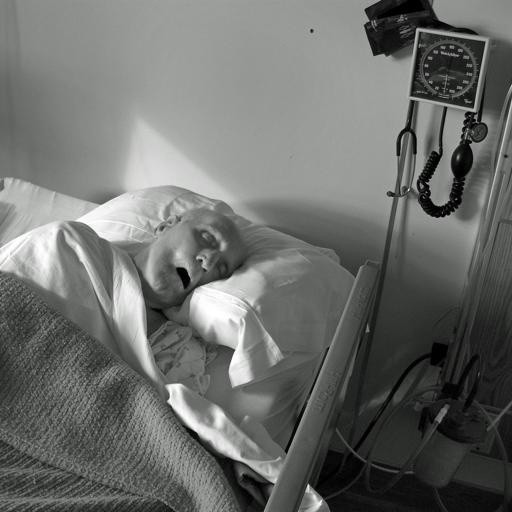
[131, 206, 249, 400]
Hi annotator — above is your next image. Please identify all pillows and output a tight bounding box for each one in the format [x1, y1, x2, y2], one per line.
[72, 184, 355, 391]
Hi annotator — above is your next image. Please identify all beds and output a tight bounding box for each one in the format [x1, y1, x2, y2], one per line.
[0, 178, 384, 512]
